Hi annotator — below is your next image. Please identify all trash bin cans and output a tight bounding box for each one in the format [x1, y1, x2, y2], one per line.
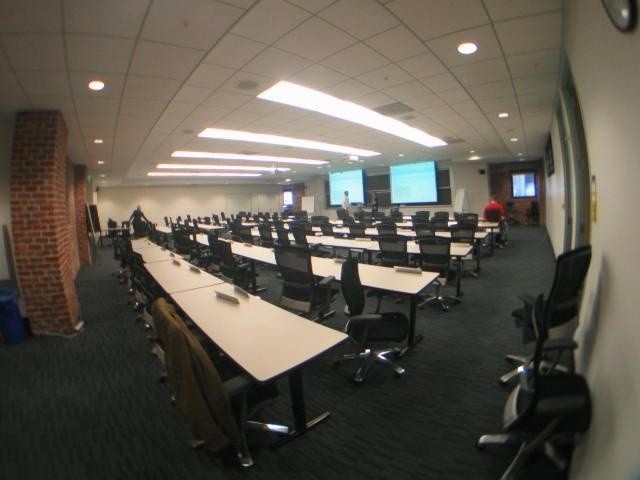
[0, 279, 28, 345]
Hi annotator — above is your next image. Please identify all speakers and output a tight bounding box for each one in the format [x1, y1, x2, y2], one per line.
[479, 169, 486, 174]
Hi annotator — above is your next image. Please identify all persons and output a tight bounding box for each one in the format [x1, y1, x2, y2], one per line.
[342, 191, 350, 212]
[108, 218, 114, 237]
[372, 192, 378, 214]
[483, 194, 508, 247]
[128, 205, 151, 232]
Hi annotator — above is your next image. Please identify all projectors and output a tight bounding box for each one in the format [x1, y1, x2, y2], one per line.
[343, 155, 359, 162]
[269, 169, 282, 175]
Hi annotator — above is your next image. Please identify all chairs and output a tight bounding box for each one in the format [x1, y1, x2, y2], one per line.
[479, 243, 592, 479]
[107, 209, 507, 468]
